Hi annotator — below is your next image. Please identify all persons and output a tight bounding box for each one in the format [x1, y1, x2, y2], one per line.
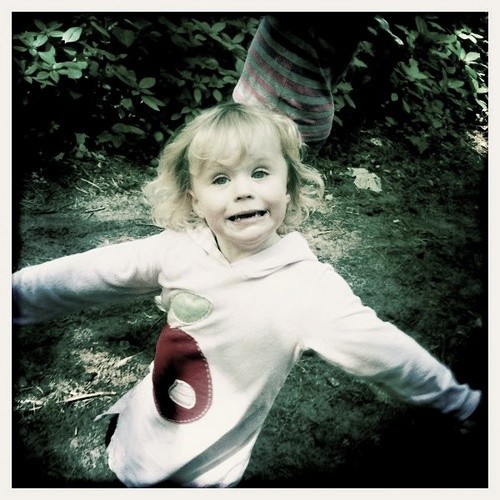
[11, 98, 487, 489]
[232, 12, 372, 172]
